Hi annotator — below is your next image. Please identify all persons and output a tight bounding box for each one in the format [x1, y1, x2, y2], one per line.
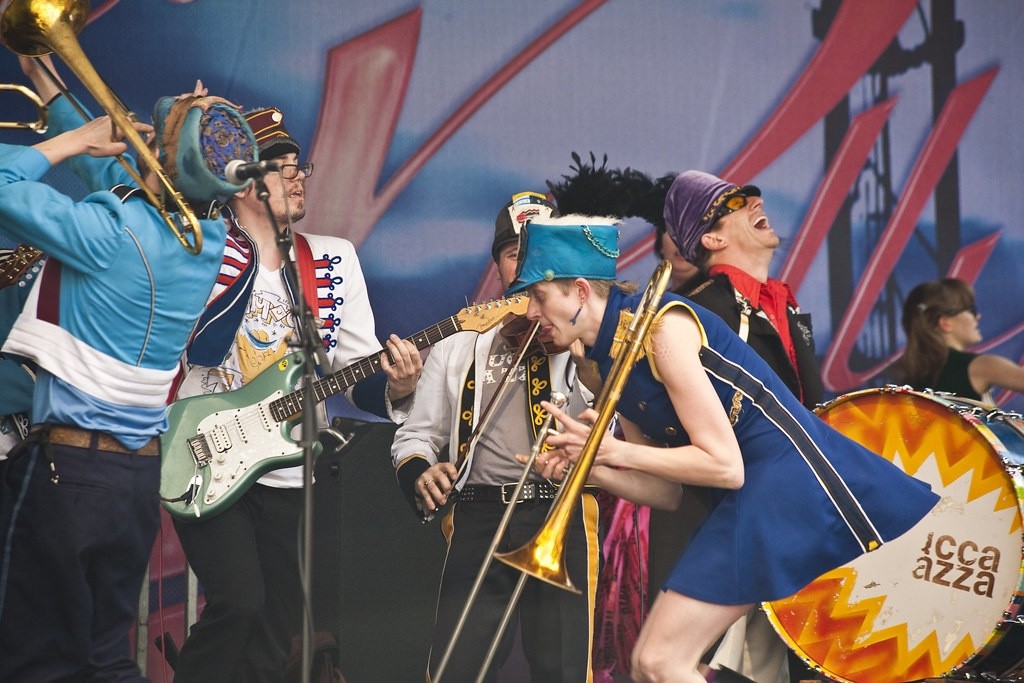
[168, 101, 423, 683]
[390, 193, 622, 683]
[888, 279, 1024, 404]
[665, 169, 828, 410]
[654, 223, 702, 293]
[502, 213, 943, 683]
[1, 54, 260, 683]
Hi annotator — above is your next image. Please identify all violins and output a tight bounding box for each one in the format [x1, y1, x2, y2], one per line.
[498, 314, 574, 357]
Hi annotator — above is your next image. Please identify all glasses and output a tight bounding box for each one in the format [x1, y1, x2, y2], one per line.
[269, 162, 314, 180]
[706, 190, 760, 233]
[946, 303, 976, 318]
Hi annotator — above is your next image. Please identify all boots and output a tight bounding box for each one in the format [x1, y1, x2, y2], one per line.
[709, 663, 757, 683]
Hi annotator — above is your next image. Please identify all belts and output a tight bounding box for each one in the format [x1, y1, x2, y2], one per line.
[27, 424, 160, 457]
[454, 481, 560, 505]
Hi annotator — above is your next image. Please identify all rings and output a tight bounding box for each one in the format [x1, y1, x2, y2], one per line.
[424, 479, 436, 485]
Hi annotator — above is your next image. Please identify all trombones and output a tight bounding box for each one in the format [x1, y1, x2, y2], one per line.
[432, 257, 676, 682]
[0, 0, 204, 256]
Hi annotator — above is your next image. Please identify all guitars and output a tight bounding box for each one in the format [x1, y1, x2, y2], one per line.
[146, 291, 532, 527]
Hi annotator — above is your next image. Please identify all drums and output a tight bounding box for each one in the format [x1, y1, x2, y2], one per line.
[756, 384, 1024, 682]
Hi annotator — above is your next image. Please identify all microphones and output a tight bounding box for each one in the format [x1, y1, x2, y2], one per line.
[223, 157, 282, 187]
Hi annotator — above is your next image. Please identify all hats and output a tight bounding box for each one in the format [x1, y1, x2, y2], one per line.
[662, 170, 761, 263]
[151, 93, 259, 206]
[503, 212, 621, 297]
[491, 191, 560, 264]
[241, 106, 301, 162]
